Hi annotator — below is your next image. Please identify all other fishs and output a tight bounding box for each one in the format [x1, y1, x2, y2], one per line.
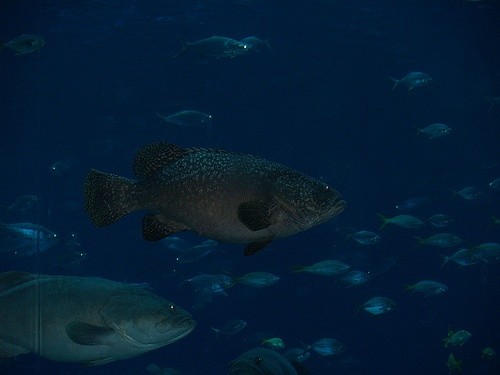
[0, 32, 500, 375]
